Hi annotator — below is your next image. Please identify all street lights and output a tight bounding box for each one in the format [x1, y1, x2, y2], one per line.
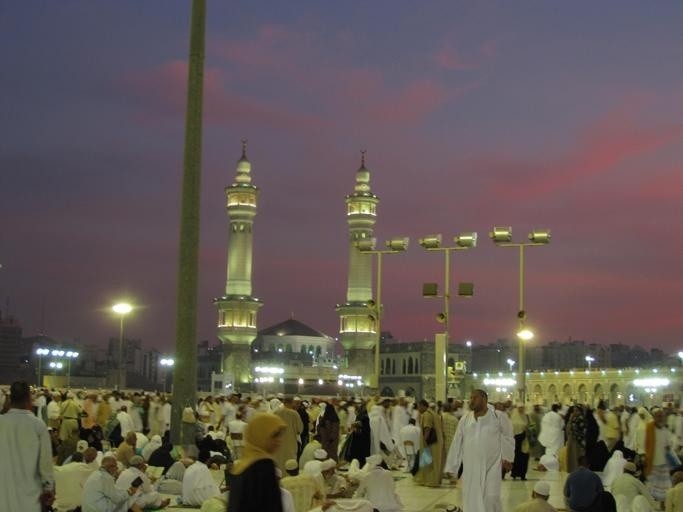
[583, 355, 596, 370]
[353, 233, 413, 392]
[34, 347, 50, 386]
[110, 301, 139, 392]
[48, 349, 66, 387]
[485, 223, 554, 408]
[65, 350, 80, 389]
[159, 358, 174, 392]
[505, 357, 516, 372]
[415, 227, 480, 407]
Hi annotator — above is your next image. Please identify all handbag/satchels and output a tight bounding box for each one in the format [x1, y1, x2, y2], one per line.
[422, 427, 439, 446]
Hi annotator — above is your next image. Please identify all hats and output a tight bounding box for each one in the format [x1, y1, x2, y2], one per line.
[276, 393, 285, 399]
[624, 462, 636, 472]
[35, 389, 385, 472]
[533, 481, 551, 496]
